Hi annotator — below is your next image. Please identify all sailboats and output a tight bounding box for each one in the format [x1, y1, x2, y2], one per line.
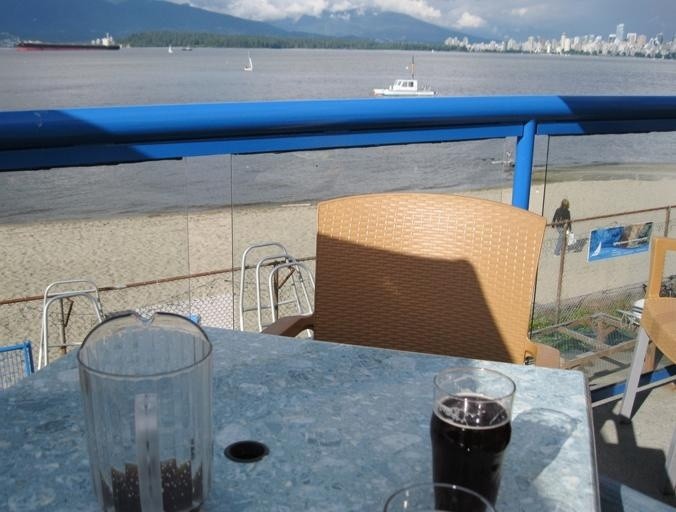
[168, 44, 173, 53]
[243, 56, 254, 72]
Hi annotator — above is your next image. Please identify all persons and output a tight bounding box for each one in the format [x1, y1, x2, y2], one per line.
[551, 199, 574, 257]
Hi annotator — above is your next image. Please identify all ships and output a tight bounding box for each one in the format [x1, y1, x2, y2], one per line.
[14, 32, 124, 52]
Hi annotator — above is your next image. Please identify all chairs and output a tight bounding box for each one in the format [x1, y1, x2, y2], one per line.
[259, 191, 561, 369]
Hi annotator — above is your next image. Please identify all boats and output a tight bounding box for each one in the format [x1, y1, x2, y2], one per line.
[180, 45, 193, 52]
[371, 77, 438, 99]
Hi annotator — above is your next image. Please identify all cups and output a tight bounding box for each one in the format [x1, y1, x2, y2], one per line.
[75, 311, 214, 511]
[428, 368, 517, 510]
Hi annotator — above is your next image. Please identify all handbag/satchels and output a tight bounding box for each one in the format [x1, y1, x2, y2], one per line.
[566, 231, 576, 246]
